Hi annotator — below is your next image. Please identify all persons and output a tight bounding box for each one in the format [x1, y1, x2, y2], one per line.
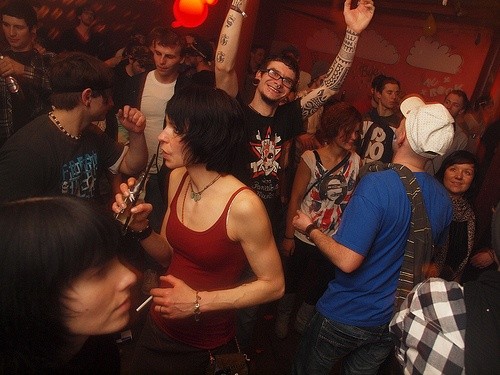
[0, 4, 57, 148]
[103, 0, 500, 375]
[294, 94, 453, 375]
[0, 195, 136, 375]
[111, 84, 285, 375]
[58, 3, 108, 58]
[0, 52, 147, 206]
[136, 27, 193, 163]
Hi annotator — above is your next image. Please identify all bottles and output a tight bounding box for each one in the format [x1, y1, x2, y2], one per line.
[0, 54, 22, 97]
[190, 43, 212, 61]
[112, 170, 152, 237]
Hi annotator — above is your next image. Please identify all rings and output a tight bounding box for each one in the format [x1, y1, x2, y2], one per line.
[160, 306, 162, 313]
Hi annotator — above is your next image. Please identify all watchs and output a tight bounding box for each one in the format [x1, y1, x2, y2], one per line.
[305, 224, 319, 242]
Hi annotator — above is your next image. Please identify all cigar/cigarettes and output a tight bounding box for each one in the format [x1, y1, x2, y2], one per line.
[136, 296, 153, 312]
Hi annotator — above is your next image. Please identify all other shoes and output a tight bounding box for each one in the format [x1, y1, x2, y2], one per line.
[142, 270, 160, 295]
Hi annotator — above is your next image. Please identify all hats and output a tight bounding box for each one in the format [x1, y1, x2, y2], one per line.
[401, 96, 455, 159]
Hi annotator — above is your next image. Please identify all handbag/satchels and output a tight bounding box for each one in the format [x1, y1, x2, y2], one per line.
[213, 352, 250, 375]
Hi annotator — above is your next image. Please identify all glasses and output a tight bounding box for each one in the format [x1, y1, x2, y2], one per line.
[261, 69, 295, 89]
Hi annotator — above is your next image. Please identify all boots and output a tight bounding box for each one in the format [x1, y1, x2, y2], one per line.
[273, 293, 296, 338]
[294, 300, 314, 335]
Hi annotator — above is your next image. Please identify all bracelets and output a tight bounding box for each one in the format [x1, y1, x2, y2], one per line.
[231, 5, 247, 18]
[194, 290, 201, 315]
[285, 236, 293, 240]
[132, 219, 154, 240]
[487, 250, 494, 260]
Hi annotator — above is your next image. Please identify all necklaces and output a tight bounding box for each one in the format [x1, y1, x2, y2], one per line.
[182, 179, 192, 222]
[190, 172, 221, 201]
[249, 104, 261, 114]
[49, 111, 82, 139]
